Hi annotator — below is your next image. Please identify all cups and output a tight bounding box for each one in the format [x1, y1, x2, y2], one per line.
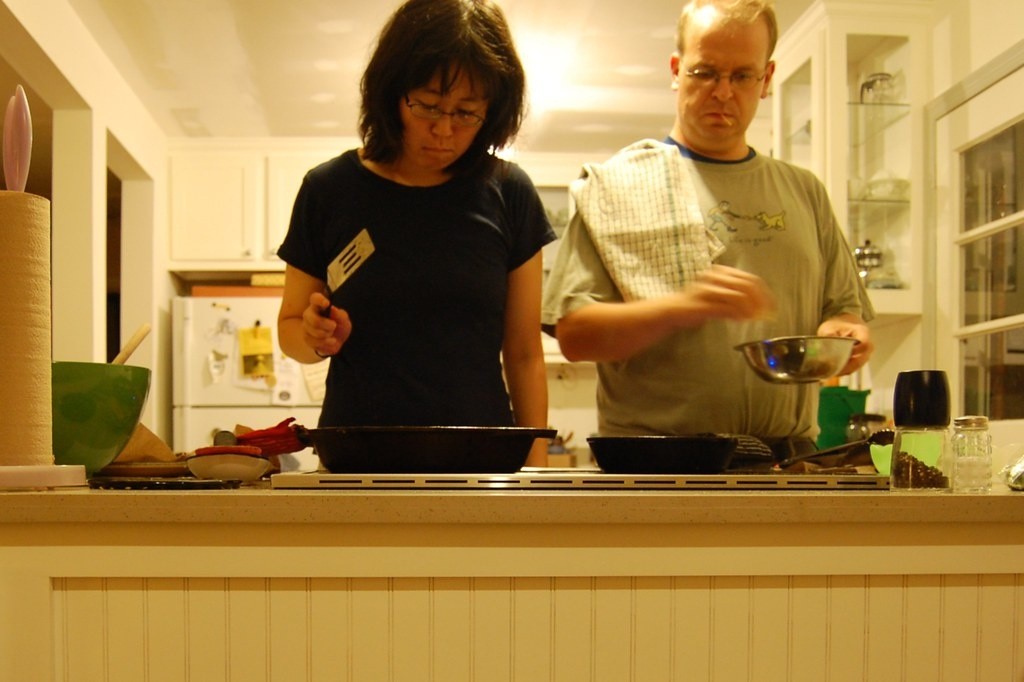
[858, 73, 893, 104]
[951, 417, 992, 493]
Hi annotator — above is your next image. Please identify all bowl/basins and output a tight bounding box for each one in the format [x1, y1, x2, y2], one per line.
[51, 361, 150, 479]
[733, 336, 860, 383]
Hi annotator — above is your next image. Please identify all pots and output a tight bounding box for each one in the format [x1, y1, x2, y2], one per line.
[293, 423, 558, 473]
[589, 434, 739, 473]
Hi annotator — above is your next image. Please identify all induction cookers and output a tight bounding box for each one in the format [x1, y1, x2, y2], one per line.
[273, 469, 890, 490]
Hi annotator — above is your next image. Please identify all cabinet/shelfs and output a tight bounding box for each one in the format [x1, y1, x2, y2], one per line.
[770, 14, 924, 317]
[534, 186, 574, 363]
[169, 150, 338, 283]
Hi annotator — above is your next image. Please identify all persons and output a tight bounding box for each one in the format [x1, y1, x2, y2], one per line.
[541, 0, 875, 465]
[277, 0, 558, 469]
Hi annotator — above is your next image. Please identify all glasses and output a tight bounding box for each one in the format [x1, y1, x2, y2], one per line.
[681, 58, 770, 90]
[403, 90, 489, 127]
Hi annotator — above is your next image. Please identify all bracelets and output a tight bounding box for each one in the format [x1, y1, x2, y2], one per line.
[315, 350, 330, 358]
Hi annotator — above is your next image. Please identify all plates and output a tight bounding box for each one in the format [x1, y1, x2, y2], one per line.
[94, 460, 193, 476]
[185, 452, 271, 485]
